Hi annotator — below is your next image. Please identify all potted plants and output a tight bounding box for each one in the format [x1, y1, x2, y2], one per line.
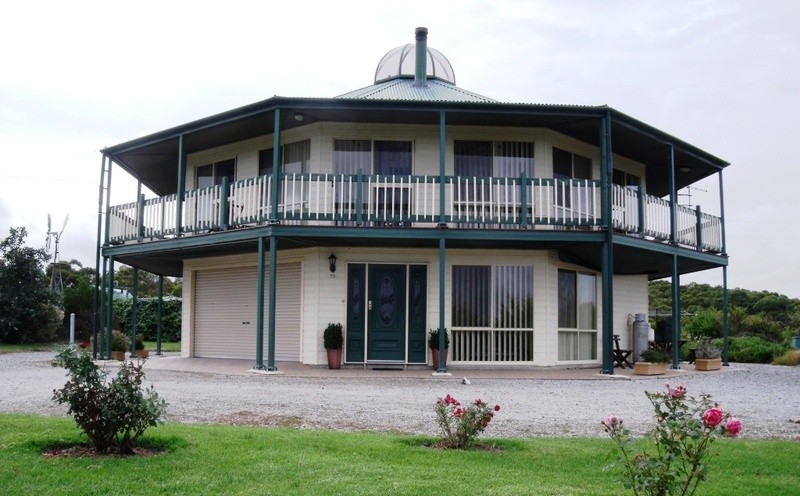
[695, 343, 722, 371]
[135, 335, 149, 358]
[635, 351, 671, 375]
[97, 327, 132, 361]
[428, 327, 450, 370]
[323, 322, 343, 370]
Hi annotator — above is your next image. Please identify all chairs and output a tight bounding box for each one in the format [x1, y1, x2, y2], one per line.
[613, 335, 633, 369]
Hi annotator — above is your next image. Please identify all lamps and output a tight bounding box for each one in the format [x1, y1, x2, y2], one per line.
[328, 252, 337, 272]
